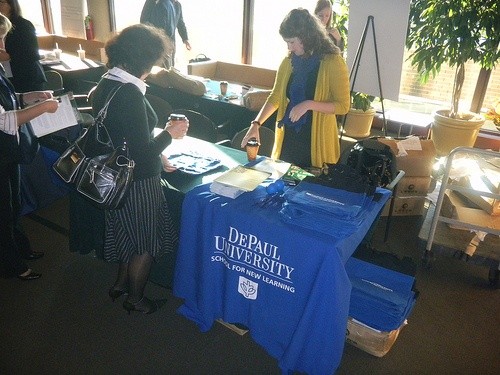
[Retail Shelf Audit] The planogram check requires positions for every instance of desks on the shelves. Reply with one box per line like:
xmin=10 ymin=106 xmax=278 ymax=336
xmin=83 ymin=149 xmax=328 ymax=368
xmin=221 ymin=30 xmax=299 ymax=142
xmin=19 ymin=127 xmax=394 ymax=374
xmin=197 ymin=81 xmax=251 ymax=147
xmin=36 ymin=50 xmax=104 ymax=91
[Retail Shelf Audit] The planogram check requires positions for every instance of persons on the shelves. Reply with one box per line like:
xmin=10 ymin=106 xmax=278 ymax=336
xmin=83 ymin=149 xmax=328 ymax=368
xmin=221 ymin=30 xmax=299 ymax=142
xmin=79 ymin=23 xmax=190 ymax=314
xmin=0 ymin=0 xmax=47 ymax=92
xmin=315 ymin=0 xmax=345 ymax=53
xmin=240 ymin=9 xmax=350 ymax=168
xmin=140 ymin=0 xmax=191 ymax=70
xmin=0 ymin=13 xmax=58 ymax=280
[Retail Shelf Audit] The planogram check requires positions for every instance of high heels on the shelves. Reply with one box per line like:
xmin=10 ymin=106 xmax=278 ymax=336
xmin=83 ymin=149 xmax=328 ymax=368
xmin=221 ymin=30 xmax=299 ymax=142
xmin=108 ymin=287 xmax=129 ymax=302
xmin=122 ymin=296 xmax=168 ymax=315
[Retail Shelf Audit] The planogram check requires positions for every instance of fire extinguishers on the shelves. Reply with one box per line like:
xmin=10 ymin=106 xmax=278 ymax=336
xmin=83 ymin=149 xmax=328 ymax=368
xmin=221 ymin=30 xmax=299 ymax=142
xmin=84 ymin=15 xmax=95 ymax=40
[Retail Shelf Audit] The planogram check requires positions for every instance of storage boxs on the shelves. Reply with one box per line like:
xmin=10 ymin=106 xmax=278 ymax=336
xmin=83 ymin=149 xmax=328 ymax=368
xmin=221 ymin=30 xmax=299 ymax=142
xmin=341 ymin=258 xmax=418 ymax=360
xmin=374 ymin=137 xmax=500 ymax=246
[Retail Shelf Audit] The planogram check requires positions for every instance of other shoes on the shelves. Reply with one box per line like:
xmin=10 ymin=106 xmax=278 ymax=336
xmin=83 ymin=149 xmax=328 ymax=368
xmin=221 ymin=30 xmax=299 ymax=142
xmin=25 ymin=250 xmax=43 ymax=259
xmin=18 ymin=270 xmax=41 ymax=280
xmin=88 ymin=248 xmax=96 ymax=257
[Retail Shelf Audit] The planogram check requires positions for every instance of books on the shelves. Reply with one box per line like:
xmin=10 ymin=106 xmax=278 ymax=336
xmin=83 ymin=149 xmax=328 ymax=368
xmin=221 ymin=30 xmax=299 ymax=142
xmin=210 ymin=164 xmax=272 ymax=199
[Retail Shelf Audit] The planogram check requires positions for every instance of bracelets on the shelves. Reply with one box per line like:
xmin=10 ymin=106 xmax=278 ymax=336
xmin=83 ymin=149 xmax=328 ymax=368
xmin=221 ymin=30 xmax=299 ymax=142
xmin=251 ymin=121 xmax=260 ymax=125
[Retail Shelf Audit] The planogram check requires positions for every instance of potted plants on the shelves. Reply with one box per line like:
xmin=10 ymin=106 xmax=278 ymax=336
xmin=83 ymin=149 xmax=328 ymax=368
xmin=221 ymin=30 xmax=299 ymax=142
xmin=406 ymin=0 xmax=500 ymax=158
xmin=339 ymin=93 xmax=381 ymax=138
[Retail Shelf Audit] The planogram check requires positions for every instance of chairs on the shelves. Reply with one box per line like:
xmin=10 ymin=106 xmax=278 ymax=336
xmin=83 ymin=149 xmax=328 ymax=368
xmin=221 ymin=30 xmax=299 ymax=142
xmin=146 ymin=90 xmax=277 ymax=166
xmin=45 ymin=70 xmax=92 ymax=116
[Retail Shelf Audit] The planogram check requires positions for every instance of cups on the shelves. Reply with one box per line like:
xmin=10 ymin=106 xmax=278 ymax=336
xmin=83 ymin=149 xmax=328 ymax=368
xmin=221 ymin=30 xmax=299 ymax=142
xmin=245 ymin=140 xmax=259 ymax=160
xmin=220 ymin=81 xmax=228 ymax=95
xmin=170 ymin=114 xmax=185 ymax=139
xmin=54 ymin=49 xmax=62 ymax=59
xmin=77 ymin=50 xmax=85 ymax=60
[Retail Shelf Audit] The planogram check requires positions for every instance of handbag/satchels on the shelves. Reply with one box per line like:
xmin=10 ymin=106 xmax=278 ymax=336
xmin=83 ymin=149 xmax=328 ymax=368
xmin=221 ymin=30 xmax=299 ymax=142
xmin=54 ymin=83 xmax=135 ymax=209
xmin=347 ymin=146 xmax=391 ymax=186
xmin=321 ymin=163 xmax=363 ymax=192
